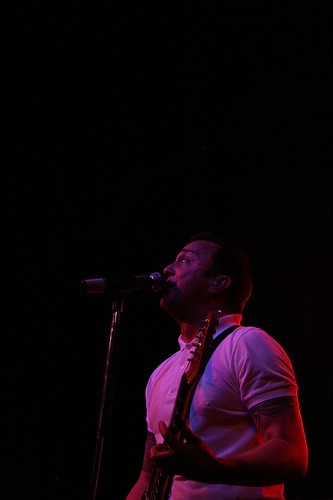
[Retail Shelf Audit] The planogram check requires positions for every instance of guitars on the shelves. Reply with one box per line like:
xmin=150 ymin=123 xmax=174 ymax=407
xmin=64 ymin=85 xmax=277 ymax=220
xmin=138 ymin=309 xmax=219 ymax=500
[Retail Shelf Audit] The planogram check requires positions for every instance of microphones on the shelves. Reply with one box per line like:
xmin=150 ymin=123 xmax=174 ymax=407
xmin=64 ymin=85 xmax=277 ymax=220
xmin=81 ymin=269 xmax=164 ymax=297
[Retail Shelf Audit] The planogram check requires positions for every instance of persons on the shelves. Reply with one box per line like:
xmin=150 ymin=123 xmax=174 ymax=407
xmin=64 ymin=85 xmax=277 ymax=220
xmin=123 ymin=231 xmax=310 ymax=500
